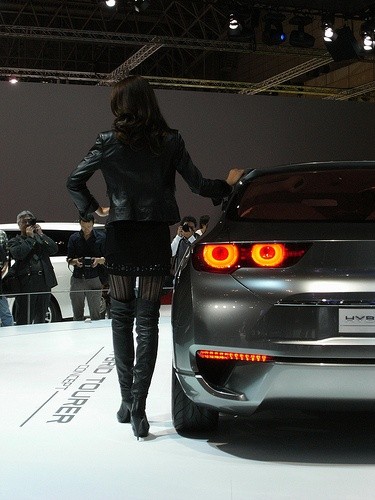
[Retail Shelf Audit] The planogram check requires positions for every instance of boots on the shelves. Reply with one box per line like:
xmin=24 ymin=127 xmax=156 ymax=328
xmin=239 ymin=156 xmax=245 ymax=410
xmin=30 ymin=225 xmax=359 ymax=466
xmin=129 ymin=300 xmax=162 ymax=438
xmin=110 ymin=296 xmax=137 ymax=422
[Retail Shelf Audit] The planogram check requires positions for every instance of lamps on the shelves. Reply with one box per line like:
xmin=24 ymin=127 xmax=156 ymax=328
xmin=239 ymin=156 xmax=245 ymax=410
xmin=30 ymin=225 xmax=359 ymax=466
xmin=271 ymin=28 xmax=286 ymax=41
xmin=360 ymin=20 xmax=375 ymax=51
xmin=228 ymin=12 xmax=241 ymax=30
xmin=321 ymin=13 xmax=334 ymax=42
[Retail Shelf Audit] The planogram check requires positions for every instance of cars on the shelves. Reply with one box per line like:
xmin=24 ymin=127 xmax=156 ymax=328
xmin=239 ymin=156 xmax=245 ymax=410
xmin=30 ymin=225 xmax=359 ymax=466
xmin=0 ymin=221 xmax=139 ymax=323
xmin=171 ymin=159 xmax=375 ymax=438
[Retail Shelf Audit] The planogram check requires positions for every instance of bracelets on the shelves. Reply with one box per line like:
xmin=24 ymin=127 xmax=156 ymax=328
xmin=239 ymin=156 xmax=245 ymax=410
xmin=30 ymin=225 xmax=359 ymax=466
xmin=40 ymin=233 xmax=44 ymax=237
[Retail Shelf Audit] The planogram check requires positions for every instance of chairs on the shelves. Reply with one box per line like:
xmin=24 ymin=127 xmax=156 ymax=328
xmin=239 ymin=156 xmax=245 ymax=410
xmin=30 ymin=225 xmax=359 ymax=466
xmin=342 ymin=188 xmax=375 ymax=222
xmin=240 ymin=192 xmax=319 ymax=219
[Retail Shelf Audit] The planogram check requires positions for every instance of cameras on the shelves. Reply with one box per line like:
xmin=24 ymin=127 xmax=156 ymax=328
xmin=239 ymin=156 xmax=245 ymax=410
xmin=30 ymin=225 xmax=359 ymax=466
xmin=80 ymin=256 xmax=94 ymax=267
xmin=182 ymin=224 xmax=190 ymax=232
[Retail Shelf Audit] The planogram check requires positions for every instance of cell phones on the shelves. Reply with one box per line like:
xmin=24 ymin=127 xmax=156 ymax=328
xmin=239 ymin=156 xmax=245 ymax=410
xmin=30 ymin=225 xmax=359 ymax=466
xmin=30 ymin=219 xmax=36 ymax=227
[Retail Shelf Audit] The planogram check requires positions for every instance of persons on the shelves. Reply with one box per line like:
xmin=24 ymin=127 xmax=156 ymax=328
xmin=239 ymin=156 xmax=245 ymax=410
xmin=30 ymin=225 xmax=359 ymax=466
xmin=195 ymin=215 xmax=210 ymax=237
xmin=9 ymin=210 xmax=58 ymax=325
xmin=67 ymin=78 xmax=242 ymax=441
xmin=0 ymin=230 xmax=14 ymax=326
xmin=67 ymin=212 xmax=106 ymax=321
xmin=170 ymin=216 xmax=200 ymax=273
